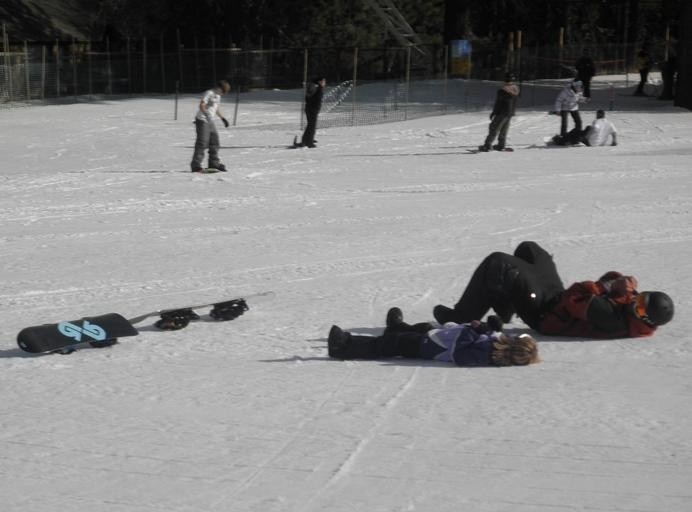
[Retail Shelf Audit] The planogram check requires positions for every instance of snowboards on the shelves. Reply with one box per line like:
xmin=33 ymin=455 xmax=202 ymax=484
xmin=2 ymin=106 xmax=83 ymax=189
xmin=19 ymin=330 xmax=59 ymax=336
xmin=17 ymin=312 xmax=139 ymax=353
xmin=193 ymin=164 xmax=228 ymax=173
xmin=289 ymin=139 xmax=318 ymax=149
xmin=466 ymin=147 xmax=514 ymax=153
xmin=127 ymin=291 xmax=274 ymax=330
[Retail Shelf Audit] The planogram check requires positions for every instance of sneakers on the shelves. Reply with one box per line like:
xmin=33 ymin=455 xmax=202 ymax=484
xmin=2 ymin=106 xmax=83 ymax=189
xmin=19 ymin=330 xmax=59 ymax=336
xmin=493 ymin=144 xmax=505 ymax=151
xmin=477 ymin=144 xmax=492 ymax=152
xmin=191 ymin=162 xmax=229 ymax=173
xmin=430 ymin=300 xmax=467 ymax=332
xmin=382 ymin=303 xmax=407 ymax=339
xmin=492 ymin=297 xmax=515 ymax=325
xmin=327 ymin=322 xmax=349 ymax=363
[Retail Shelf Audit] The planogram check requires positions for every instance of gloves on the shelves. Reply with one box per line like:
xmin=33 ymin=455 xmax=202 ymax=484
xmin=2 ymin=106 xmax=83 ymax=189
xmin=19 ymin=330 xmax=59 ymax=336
xmin=204 ymin=113 xmax=231 ymax=129
xmin=612 ymin=273 xmax=636 ymax=305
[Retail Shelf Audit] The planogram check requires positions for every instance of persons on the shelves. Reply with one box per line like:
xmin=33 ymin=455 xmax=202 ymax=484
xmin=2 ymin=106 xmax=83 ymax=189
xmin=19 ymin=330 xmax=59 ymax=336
xmin=654 ymin=40 xmax=677 ymax=100
xmin=479 ymin=73 xmax=519 ymax=152
xmin=553 ymin=110 xmax=618 ymax=146
xmin=632 ymin=44 xmax=654 ymax=96
xmin=191 ymin=79 xmax=231 ymax=172
xmin=575 ymin=49 xmax=596 ymax=102
xmin=433 ymin=241 xmax=674 ymax=338
xmin=555 ymin=79 xmax=591 ymax=136
xmin=302 ymin=76 xmax=327 ymax=148
xmin=328 ymin=307 xmax=539 ymax=366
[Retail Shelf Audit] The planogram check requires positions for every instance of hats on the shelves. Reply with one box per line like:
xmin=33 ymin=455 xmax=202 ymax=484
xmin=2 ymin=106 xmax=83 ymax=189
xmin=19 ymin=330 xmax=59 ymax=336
xmin=504 ymin=71 xmax=517 ymax=83
xmin=219 ymin=80 xmax=232 ymax=93
xmin=572 ymin=80 xmax=585 ymax=92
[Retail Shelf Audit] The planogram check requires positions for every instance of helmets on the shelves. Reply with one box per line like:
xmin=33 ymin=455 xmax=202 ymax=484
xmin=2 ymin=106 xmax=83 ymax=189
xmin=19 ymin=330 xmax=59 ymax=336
xmin=631 ymin=289 xmax=674 ymax=327
